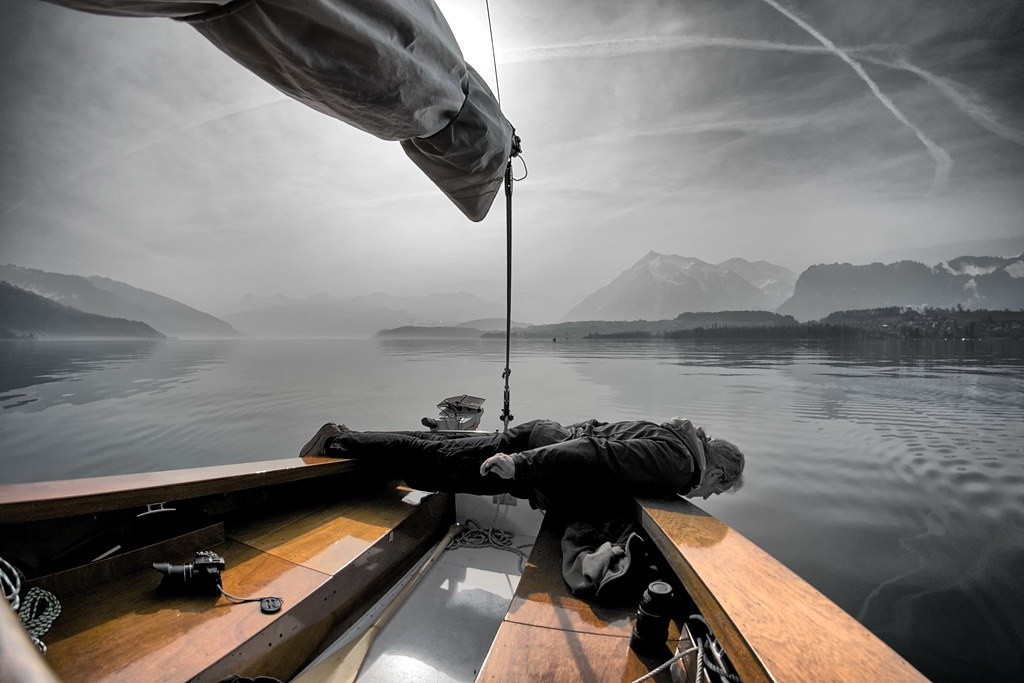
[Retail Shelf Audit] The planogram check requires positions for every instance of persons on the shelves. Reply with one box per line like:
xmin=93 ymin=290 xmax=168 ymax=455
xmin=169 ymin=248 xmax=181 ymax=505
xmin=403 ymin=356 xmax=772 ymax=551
xmin=298 ymin=414 xmax=746 ymax=501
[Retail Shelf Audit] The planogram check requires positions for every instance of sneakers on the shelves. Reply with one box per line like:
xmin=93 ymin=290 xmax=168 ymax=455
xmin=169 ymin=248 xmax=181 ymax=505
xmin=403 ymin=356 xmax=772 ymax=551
xmin=299 ymin=422 xmax=349 ymax=457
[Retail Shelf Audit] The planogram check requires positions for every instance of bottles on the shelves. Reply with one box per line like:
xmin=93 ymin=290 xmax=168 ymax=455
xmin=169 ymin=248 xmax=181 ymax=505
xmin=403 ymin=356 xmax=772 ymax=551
xmin=629 ymin=582 xmax=673 ymax=656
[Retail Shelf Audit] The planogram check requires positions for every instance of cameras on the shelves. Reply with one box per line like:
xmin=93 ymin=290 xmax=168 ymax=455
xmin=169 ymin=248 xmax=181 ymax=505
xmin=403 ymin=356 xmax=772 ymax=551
xmin=153 ymin=551 xmax=225 ymax=600
